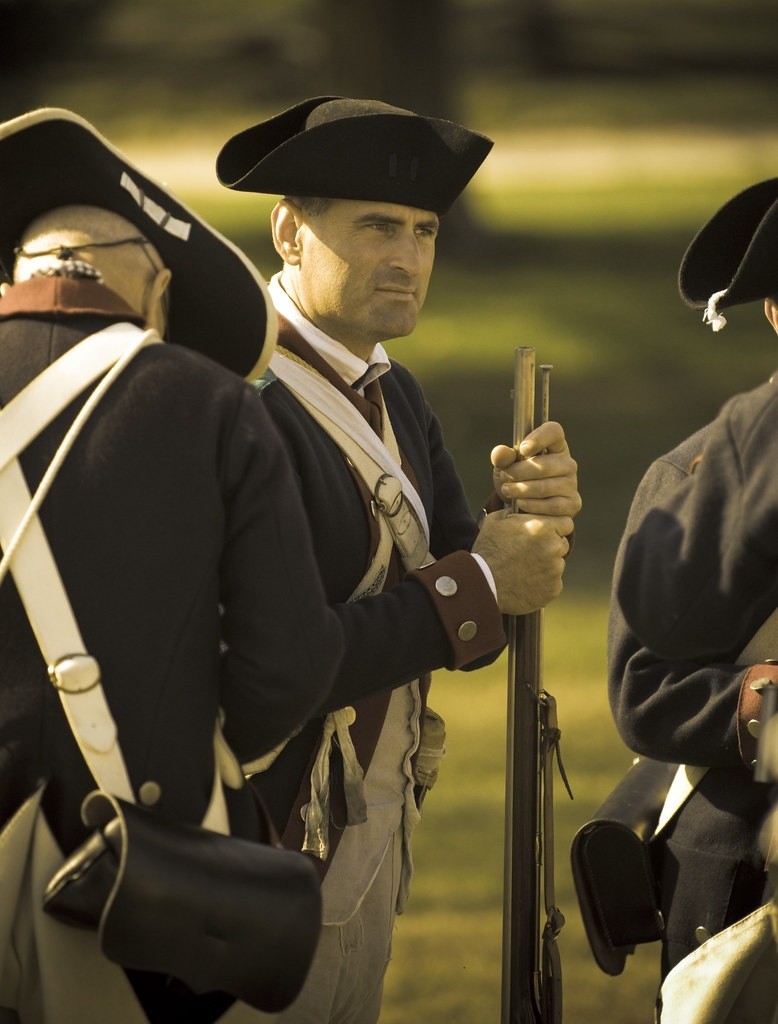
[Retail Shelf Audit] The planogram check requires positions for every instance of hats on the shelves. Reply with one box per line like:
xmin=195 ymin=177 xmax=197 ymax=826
xmin=0 ymin=105 xmax=279 ymax=382
xmin=215 ymin=93 xmax=495 ymax=216
xmin=679 ymin=176 xmax=778 ymax=333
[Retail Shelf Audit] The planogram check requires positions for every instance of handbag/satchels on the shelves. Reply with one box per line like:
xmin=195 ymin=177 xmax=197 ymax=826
xmin=571 ymin=754 xmax=685 ymax=975
xmin=40 ymin=790 xmax=323 ymax=1010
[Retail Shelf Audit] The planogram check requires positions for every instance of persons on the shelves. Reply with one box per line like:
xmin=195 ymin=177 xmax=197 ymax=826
xmin=608 ymin=177 xmax=778 ymax=1024
xmin=1 ymin=106 xmax=344 ymax=1024
xmin=220 ymin=96 xmax=581 ymax=1022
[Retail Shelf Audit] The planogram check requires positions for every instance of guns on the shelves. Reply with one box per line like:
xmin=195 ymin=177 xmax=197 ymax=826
xmin=497 ymin=346 xmax=566 ymax=1024
